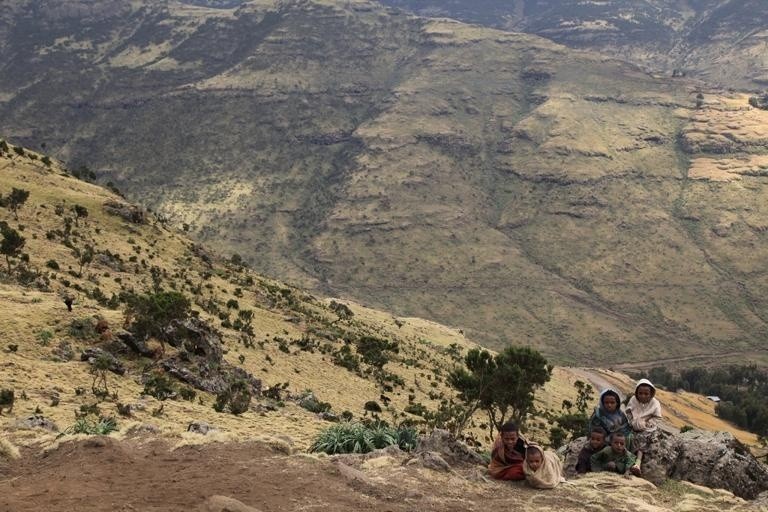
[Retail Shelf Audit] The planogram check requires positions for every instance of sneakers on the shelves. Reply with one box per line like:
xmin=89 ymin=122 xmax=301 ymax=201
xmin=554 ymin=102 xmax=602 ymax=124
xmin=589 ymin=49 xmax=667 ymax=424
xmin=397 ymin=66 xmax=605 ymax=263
xmin=631 ymin=465 xmax=641 ymax=476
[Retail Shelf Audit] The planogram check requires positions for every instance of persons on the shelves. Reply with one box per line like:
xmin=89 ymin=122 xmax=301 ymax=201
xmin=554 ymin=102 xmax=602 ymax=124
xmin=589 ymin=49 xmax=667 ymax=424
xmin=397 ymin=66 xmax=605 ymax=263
xmin=486 ymin=421 xmax=527 ymax=481
xmin=521 ymin=443 xmax=566 ymax=490
xmin=625 ymin=378 xmax=663 ymax=475
xmin=590 ymin=432 xmax=637 ymax=476
xmin=587 ymin=387 xmax=633 ymax=450
xmin=574 ymin=427 xmax=608 ymax=473
